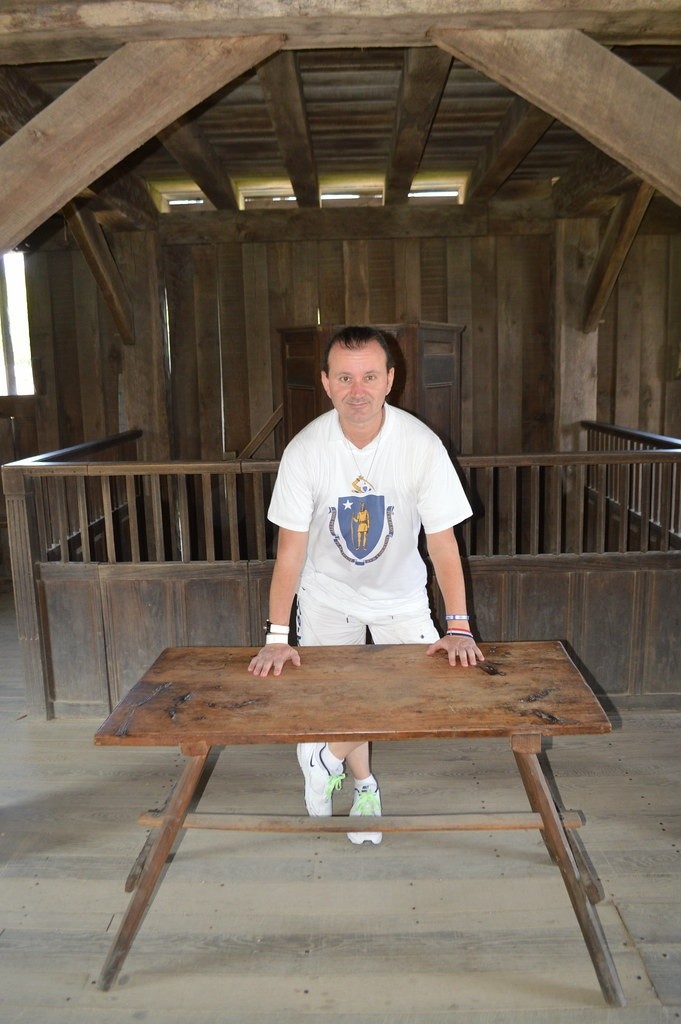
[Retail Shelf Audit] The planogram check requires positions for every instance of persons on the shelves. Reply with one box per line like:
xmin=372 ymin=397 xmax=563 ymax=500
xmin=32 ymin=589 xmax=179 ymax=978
xmin=248 ymin=327 xmax=486 ymax=844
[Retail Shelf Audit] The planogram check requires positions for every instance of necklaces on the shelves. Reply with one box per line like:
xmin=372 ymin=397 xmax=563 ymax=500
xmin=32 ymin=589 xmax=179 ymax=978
xmin=339 ymin=414 xmax=384 ymax=493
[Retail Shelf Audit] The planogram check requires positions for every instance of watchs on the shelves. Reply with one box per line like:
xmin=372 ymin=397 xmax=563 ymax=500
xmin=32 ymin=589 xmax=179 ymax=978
xmin=263 ymin=619 xmax=290 ymax=635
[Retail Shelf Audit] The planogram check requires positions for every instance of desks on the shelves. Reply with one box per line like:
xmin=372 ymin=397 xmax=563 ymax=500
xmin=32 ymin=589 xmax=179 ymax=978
xmin=91 ymin=639 xmax=626 ymax=1007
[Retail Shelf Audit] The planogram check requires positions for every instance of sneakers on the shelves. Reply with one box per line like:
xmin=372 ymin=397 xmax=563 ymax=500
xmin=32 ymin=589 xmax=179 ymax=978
xmin=297 ymin=742 xmax=345 ymax=816
xmin=347 ymin=787 xmax=383 ymax=848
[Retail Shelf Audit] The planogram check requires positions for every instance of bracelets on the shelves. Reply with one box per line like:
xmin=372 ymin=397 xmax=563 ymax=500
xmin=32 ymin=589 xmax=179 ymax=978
xmin=265 ymin=635 xmax=288 ymax=645
xmin=445 ymin=628 xmax=474 ymax=638
xmin=446 ymin=615 xmax=469 ymax=620
